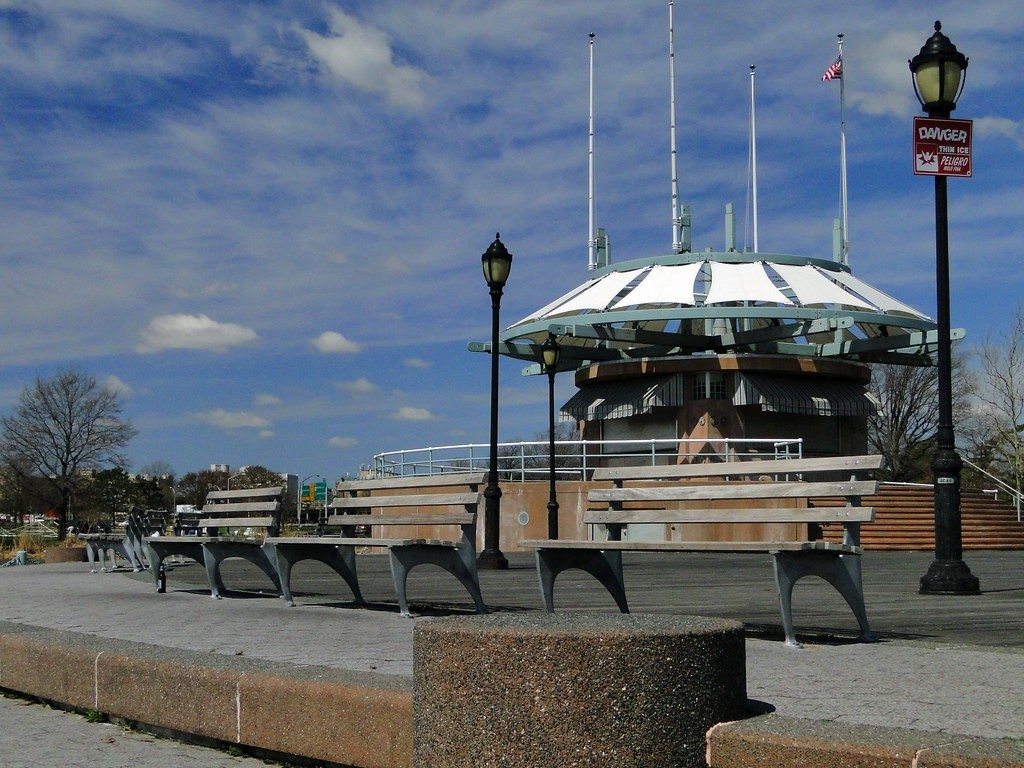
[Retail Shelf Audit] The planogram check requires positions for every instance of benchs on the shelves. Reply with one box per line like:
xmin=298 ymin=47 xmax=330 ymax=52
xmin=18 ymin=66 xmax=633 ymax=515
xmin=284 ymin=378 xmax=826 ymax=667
xmin=142 ymin=486 xmax=287 ymax=599
xmin=265 ymin=472 xmax=487 ymax=618
xmin=76 ymin=505 xmax=146 ymax=572
xmin=141 ymin=508 xmax=168 ymax=569
xmin=517 ymin=454 xmax=886 ymax=646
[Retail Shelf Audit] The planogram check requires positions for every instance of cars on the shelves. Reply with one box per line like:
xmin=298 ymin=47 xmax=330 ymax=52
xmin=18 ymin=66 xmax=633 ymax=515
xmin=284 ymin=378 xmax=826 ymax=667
xmin=119 ymin=521 xmax=130 ymax=527
xmin=174 ymin=512 xmax=208 ymax=536
xmin=355 ymin=526 xmax=367 ymax=532
xmin=111 ymin=517 xmax=124 ymax=526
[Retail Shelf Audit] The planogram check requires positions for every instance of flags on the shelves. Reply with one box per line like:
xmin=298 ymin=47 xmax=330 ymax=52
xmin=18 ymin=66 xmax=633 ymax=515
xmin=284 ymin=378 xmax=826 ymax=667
xmin=821 ymin=54 xmax=842 ymax=81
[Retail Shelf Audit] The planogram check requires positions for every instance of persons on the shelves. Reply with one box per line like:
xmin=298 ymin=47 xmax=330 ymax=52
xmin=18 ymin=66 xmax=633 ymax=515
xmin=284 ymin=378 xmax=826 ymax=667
xmin=80 ymin=521 xmax=89 ymax=533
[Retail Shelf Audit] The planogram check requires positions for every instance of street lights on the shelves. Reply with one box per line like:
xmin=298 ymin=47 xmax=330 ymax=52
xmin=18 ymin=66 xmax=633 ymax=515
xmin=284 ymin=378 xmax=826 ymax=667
xmin=170 ymin=487 xmax=176 ymax=524
xmin=540 ymin=332 xmax=562 ymax=540
xmin=298 ymin=475 xmax=319 ymax=530
xmin=227 ymin=473 xmax=246 ymax=534
xmin=247 ymin=484 xmax=262 ymax=518
xmin=909 ymin=21 xmax=983 ymax=595
xmin=477 ymin=231 xmax=514 ymax=570
